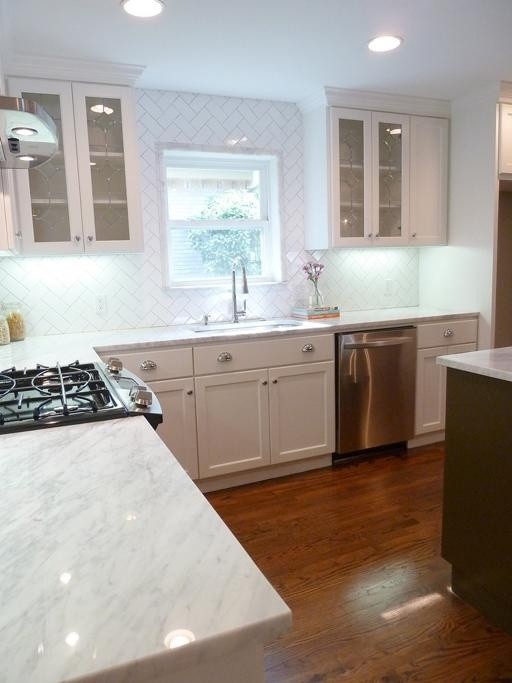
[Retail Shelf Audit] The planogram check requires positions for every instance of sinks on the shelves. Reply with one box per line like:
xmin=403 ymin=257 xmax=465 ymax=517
xmin=187 ymin=320 xmax=305 ymax=333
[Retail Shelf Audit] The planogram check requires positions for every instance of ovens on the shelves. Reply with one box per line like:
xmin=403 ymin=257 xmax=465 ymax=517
xmin=333 ymin=326 xmax=417 ymax=469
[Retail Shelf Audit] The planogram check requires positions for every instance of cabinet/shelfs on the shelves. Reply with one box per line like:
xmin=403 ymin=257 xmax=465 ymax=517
xmin=297 ymin=85 xmax=451 ymax=249
xmin=1 ymin=53 xmax=146 ymax=255
xmin=99 ymin=318 xmax=480 ymax=502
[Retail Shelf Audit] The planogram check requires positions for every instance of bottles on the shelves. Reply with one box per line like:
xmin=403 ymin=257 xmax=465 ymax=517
xmin=0 ymin=301 xmax=25 ymax=346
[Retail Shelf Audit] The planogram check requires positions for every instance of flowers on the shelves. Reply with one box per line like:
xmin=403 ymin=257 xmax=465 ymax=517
xmin=303 ymin=260 xmax=327 ymax=304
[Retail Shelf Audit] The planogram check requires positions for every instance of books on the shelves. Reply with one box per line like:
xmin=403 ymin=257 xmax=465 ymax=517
xmin=292 ymin=305 xmax=340 ymax=319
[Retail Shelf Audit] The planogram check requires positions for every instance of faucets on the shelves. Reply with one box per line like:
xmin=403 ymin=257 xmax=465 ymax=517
xmin=231 ymin=256 xmax=248 ymax=320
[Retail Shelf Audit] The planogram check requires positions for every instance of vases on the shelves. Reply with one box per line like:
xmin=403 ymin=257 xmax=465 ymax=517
xmin=308 ymin=290 xmax=324 ymax=310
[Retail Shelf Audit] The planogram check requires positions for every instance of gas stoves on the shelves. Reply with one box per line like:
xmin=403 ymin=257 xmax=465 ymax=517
xmin=0 ymin=357 xmax=163 ymax=435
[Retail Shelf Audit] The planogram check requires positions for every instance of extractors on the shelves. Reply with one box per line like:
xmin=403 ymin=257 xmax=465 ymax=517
xmin=0 ymin=97 xmax=59 ymax=169
